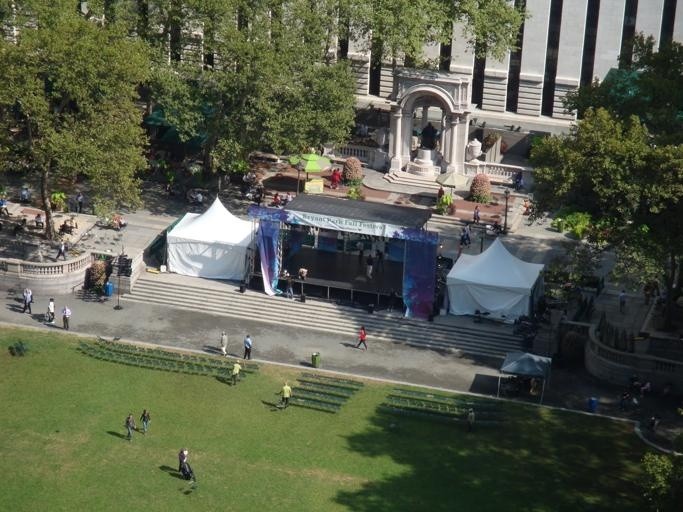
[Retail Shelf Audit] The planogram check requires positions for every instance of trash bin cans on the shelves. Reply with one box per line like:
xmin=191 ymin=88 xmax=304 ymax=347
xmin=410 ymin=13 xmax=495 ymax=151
xmin=588 ymin=396 xmax=598 ymax=409
xmin=104 ymin=282 xmax=113 ymax=296
xmin=312 ymin=352 xmax=321 ymax=368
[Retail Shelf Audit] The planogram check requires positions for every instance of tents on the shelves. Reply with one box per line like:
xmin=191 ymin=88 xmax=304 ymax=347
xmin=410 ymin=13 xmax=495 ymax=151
xmin=167 ymin=197 xmax=259 ymax=281
xmin=446 ymin=237 xmax=546 ymax=325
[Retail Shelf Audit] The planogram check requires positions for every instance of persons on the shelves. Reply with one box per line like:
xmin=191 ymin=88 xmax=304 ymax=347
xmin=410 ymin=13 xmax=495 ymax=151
xmin=514 ymin=293 xmax=635 ymax=355
xmin=220 ymin=331 xmax=252 ymax=385
xmin=188 ymin=171 xmax=292 ymax=205
xmin=459 ymin=205 xmax=481 ymax=250
xmin=330 ymin=167 xmax=345 ymax=188
xmin=179 ymin=448 xmax=196 ymax=481
xmin=366 ymin=254 xmax=376 ymax=280
xmin=467 ymin=408 xmax=476 ymax=434
xmin=337 ymin=232 xmax=386 ymax=260
xmin=127 ymin=409 xmax=151 ymax=439
xmin=22 ymin=289 xmax=72 ymax=331
xmin=619 ymin=374 xmax=675 ymax=410
xmin=354 ymin=326 xmax=368 ymax=349
xmin=285 ymin=267 xmax=308 ymax=298
xmin=276 ymin=381 xmax=292 ymax=406
xmin=2 ymin=191 xmax=84 ymax=262
xmin=619 ymin=289 xmax=627 ymax=312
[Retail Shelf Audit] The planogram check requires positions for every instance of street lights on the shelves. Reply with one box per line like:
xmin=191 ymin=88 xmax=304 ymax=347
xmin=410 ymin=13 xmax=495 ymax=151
xmin=294 ymin=159 xmax=302 ymax=196
xmin=479 ymin=227 xmax=486 ymax=252
xmin=503 ymin=187 xmax=511 ymax=238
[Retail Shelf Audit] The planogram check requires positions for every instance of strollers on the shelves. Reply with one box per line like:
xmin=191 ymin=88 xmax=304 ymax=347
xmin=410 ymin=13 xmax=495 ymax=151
xmin=180 ymin=462 xmax=199 ymax=483
